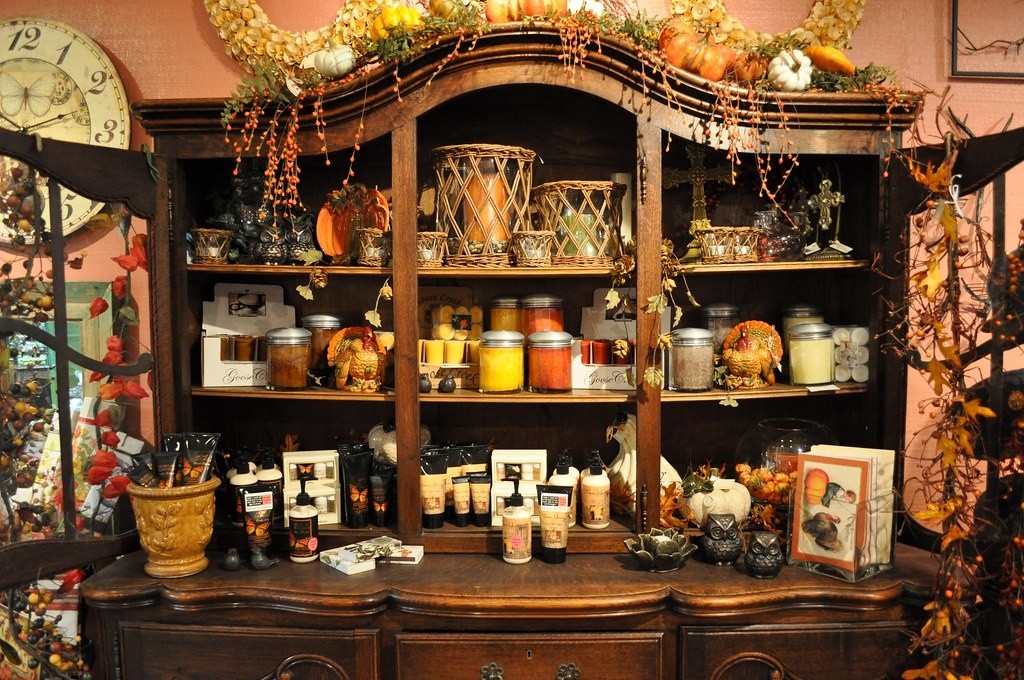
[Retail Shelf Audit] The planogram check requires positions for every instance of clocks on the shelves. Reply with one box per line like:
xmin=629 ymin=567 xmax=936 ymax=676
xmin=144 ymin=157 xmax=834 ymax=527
xmin=0 ymin=13 xmax=131 ymax=250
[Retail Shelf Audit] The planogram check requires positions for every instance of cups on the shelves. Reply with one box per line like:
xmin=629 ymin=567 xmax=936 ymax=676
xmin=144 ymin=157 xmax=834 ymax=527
xmin=220 ymin=334 xmax=268 ymax=360
xmin=581 ymin=339 xmax=632 ymax=365
xmin=418 ymin=339 xmax=480 ymax=364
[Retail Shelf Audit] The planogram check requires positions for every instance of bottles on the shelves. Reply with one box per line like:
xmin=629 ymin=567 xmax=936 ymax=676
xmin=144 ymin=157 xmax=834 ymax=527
xmin=265 ymin=326 xmax=313 ymax=388
xmin=788 ymin=323 xmax=834 ymax=384
xmin=783 ymin=306 xmax=824 ymax=354
xmin=478 ymin=329 xmax=525 ymax=393
xmin=668 ymin=328 xmax=714 ymax=391
xmin=302 ymin=315 xmax=341 ymax=378
xmin=702 ymin=303 xmax=742 ymax=353
xmin=522 ymin=295 xmax=564 ymax=330
xmin=491 ymin=299 xmax=521 ymax=330
xmin=528 ymin=330 xmax=572 ymax=392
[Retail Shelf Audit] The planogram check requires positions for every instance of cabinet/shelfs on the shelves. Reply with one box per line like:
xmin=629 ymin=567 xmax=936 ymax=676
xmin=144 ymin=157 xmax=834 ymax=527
xmin=134 ymin=22 xmax=929 ymax=556
xmin=76 ymin=543 xmax=981 ymax=680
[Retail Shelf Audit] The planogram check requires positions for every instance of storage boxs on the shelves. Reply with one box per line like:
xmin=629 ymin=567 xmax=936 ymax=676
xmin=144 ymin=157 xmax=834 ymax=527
xmin=31 ymin=396 xmax=144 ymax=535
xmin=28 ymin=578 xmax=81 ymax=647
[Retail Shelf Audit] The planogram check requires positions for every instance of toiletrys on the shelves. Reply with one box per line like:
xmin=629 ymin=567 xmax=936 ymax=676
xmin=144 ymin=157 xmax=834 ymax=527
xmin=126 ymin=432 xmax=611 ymax=564
